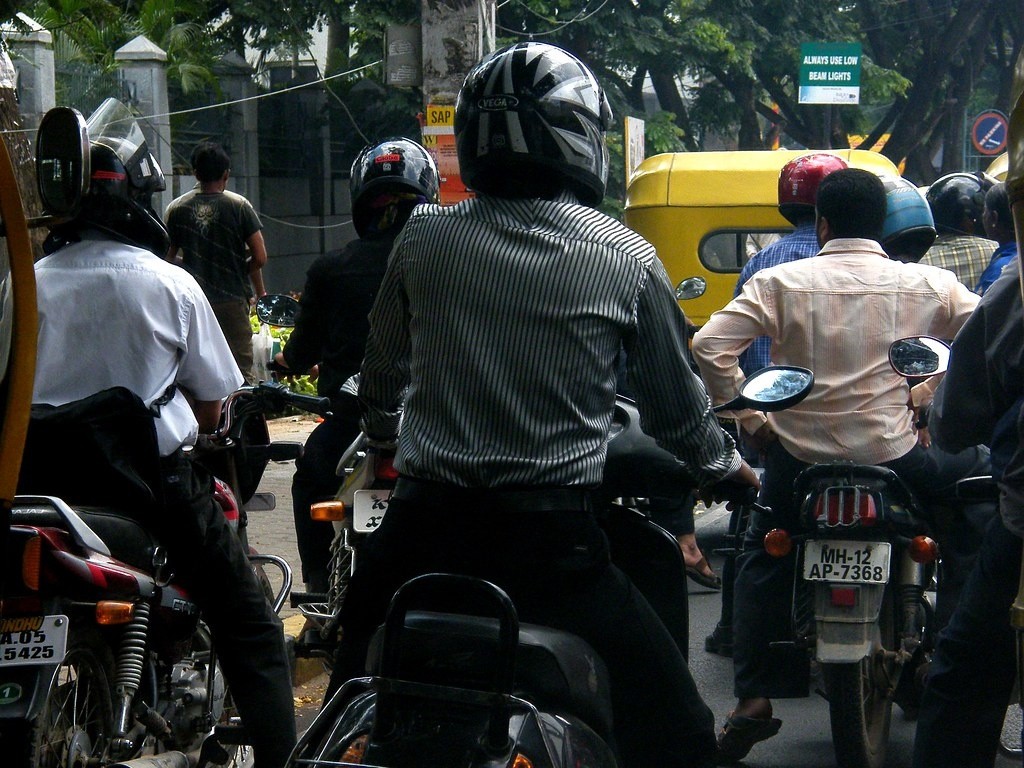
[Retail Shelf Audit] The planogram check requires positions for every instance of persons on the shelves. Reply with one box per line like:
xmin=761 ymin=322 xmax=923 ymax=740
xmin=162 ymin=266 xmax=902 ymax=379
xmin=693 ymin=154 xmax=1024 ymax=768
xmin=163 ymin=141 xmax=266 ymax=387
xmin=322 ymin=41 xmax=761 ymax=768
xmin=272 ymin=137 xmax=441 ymax=656
xmin=1 ymin=99 xmax=296 ymax=767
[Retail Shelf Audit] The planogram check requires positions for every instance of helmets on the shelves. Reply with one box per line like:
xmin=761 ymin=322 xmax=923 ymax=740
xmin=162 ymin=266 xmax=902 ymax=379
xmin=778 ymin=153 xmax=849 ymax=227
xmin=49 ymin=136 xmax=170 ymax=260
xmin=453 ymin=42 xmax=617 ymax=209
xmin=878 ymin=176 xmax=937 ymax=263
xmin=925 ymin=172 xmax=988 ymax=237
xmin=349 ymin=136 xmax=440 ymax=239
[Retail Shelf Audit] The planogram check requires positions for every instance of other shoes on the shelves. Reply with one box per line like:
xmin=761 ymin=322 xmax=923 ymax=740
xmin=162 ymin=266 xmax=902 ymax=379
xmin=705 ymin=624 xmax=735 ymax=656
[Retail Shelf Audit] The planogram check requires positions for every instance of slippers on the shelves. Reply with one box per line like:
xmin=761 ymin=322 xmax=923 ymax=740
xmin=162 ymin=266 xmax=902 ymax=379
xmin=684 ymin=556 xmax=721 ymax=590
xmin=717 ymin=711 xmax=781 ymax=761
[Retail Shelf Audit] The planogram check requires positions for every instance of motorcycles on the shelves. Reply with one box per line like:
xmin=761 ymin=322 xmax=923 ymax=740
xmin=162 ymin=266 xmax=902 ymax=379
xmin=1 ymin=378 xmax=334 ymax=767
xmin=286 ymin=364 xmax=814 ymax=767
xmin=0 ymin=109 xmax=90 ymax=602
xmin=252 ymin=148 xmax=1024 ymax=767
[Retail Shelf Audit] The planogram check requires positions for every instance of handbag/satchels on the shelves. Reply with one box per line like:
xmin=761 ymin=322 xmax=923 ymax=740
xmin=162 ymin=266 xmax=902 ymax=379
xmin=15 ymin=386 xmax=164 ymax=511
